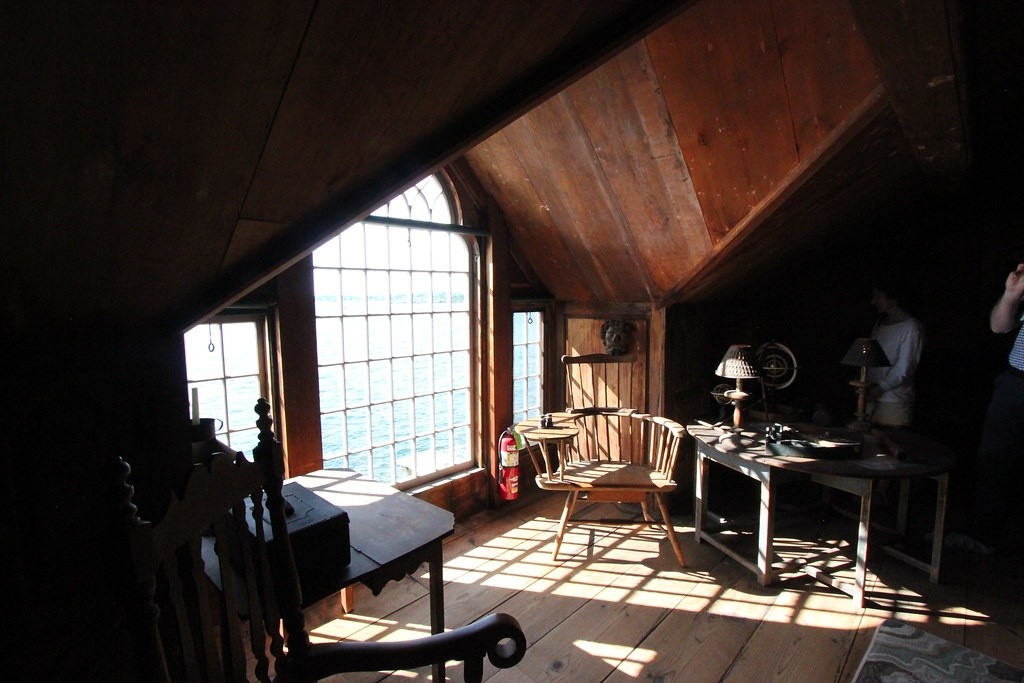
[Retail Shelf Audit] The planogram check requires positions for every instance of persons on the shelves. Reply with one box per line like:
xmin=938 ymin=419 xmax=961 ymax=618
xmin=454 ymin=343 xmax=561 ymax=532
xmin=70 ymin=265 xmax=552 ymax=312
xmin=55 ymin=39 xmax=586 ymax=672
xmin=600 ymin=321 xmax=635 ymax=356
xmin=980 ymin=264 xmax=1024 ymax=519
xmin=866 ymin=285 xmax=925 ymax=428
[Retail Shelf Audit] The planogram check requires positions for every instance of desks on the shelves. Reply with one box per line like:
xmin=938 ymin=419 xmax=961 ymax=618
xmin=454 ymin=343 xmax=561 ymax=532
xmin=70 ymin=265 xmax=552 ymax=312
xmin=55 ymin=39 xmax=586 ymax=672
xmin=689 ymin=424 xmax=947 ymax=609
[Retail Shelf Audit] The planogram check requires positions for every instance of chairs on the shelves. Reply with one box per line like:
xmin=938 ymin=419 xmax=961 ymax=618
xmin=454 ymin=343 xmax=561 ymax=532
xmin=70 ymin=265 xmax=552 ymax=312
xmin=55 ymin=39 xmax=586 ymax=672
xmin=113 ymin=395 xmax=526 ymax=683
xmin=532 ymin=350 xmax=685 ymax=571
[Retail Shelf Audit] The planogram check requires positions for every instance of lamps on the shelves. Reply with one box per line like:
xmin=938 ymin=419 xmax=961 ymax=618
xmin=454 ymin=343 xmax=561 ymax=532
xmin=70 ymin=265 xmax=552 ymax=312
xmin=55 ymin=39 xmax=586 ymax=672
xmin=714 ymin=343 xmax=759 ymax=436
xmin=837 ymin=336 xmax=892 ymax=435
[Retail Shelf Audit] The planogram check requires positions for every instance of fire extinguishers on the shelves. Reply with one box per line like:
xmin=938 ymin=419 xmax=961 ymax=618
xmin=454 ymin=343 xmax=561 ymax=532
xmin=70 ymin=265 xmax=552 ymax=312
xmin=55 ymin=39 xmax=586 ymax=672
xmin=497 ymin=422 xmax=520 ymax=501
